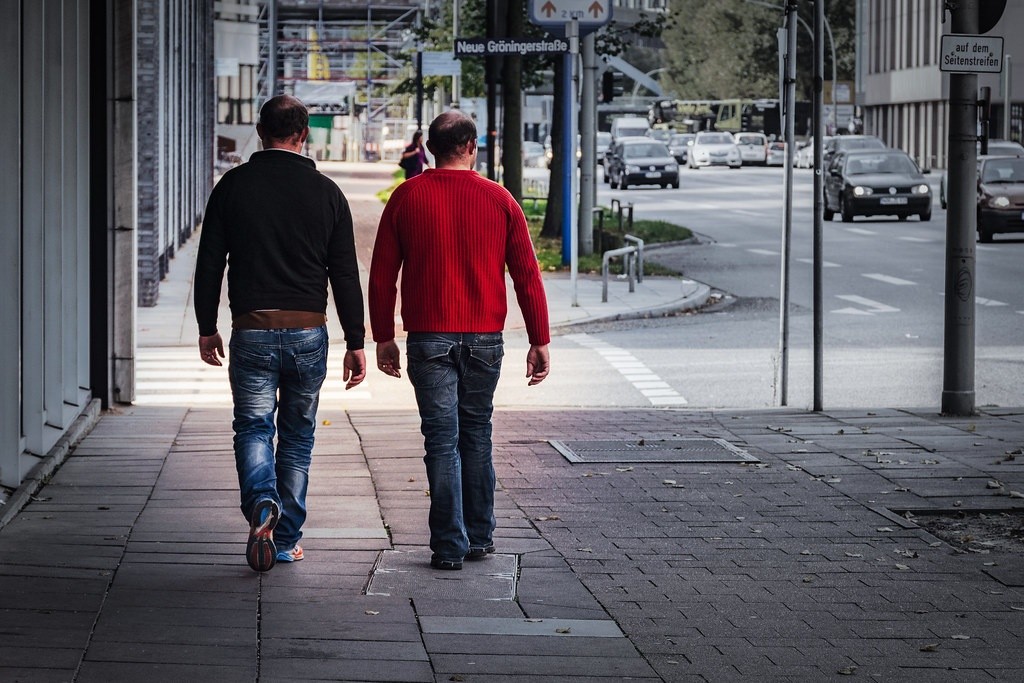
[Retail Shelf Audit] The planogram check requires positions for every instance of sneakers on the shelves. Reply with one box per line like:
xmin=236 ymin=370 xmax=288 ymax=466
xmin=277 ymin=544 xmax=303 ymax=562
xmin=246 ymin=498 xmax=281 ymax=572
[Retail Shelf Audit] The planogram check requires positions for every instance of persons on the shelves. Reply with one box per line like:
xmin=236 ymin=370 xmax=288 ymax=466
xmin=194 ymin=95 xmax=366 ymax=570
xmin=403 ymin=130 xmax=430 ymax=179
xmin=369 ymin=110 xmax=549 ymax=568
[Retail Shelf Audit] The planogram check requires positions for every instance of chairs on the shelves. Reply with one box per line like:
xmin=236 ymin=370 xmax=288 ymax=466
xmin=848 ymin=161 xmax=861 ymax=172
xmin=878 ymin=160 xmax=888 ymax=173
xmin=986 ymin=169 xmax=1001 ymax=180
xmin=1010 ymin=167 xmax=1024 ymax=179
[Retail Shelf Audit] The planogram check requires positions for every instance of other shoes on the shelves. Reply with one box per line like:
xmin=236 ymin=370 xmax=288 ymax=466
xmin=467 ymin=543 xmax=497 ymax=558
xmin=430 ymin=557 xmax=463 ymax=571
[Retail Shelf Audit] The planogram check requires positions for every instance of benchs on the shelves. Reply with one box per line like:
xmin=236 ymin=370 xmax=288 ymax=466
xmin=862 ymin=163 xmax=879 ymax=172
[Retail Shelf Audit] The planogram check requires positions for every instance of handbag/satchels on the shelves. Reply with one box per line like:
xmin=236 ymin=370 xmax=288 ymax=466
xmin=399 ymin=155 xmax=413 ymax=170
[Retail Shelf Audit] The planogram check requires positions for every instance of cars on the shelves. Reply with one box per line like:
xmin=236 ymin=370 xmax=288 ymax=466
xmin=524 ymin=117 xmax=834 ymax=170
xmin=938 ymin=137 xmax=1024 ymax=210
xmin=608 ymin=140 xmax=681 ymax=191
xmin=686 ymin=131 xmax=742 ymax=170
xmin=601 ymin=135 xmax=655 ymax=183
xmin=979 ymin=154 xmax=1024 ymax=244
xmin=823 ymin=147 xmax=935 ymax=224
xmin=822 ymin=135 xmax=890 ymax=170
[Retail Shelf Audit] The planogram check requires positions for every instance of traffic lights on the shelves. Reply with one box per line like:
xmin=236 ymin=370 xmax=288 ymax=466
xmin=601 ymin=70 xmax=626 ymax=104
